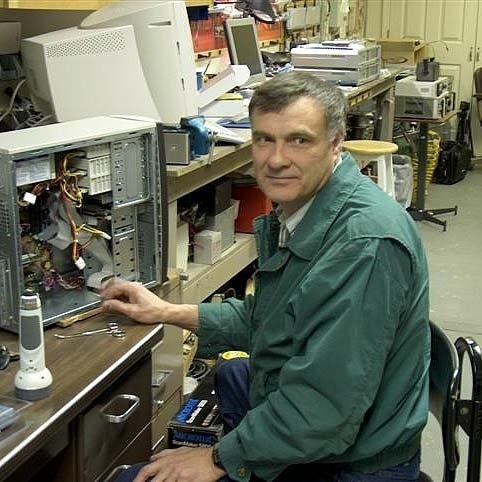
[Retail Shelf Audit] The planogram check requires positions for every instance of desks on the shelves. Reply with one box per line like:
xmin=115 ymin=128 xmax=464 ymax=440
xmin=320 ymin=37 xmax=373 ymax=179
xmin=0 ymin=315 xmax=164 ymax=482
xmin=348 ymin=100 xmax=459 ymax=232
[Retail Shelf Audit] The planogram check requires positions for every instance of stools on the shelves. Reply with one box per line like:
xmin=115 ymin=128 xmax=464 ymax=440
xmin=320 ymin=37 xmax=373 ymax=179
xmin=343 ymin=139 xmax=398 ymax=201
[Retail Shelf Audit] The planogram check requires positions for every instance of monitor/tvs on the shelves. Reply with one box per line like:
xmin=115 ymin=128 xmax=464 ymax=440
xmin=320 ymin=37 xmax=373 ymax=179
xmin=22 ymin=0 xmax=203 ymax=127
xmin=223 ymin=17 xmax=267 ymax=87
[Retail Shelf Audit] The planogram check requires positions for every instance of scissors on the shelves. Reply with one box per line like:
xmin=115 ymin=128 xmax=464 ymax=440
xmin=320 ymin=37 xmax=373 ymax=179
xmin=53 ymin=322 xmax=127 ymax=339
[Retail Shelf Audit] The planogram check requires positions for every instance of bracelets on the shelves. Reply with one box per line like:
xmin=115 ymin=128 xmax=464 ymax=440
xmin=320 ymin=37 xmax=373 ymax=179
xmin=212 ymin=444 xmax=228 ymax=473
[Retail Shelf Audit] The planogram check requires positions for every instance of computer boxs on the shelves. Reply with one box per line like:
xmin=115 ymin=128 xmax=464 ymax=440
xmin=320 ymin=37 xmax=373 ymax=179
xmin=0 ymin=112 xmax=169 ymax=335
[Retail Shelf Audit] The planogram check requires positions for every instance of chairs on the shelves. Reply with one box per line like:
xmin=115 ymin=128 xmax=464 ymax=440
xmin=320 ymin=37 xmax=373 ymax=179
xmin=419 ymin=320 xmax=482 ymax=482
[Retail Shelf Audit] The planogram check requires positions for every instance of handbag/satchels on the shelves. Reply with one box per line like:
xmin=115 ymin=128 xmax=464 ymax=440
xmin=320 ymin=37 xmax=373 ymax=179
xmin=437 ymin=141 xmax=466 ymax=184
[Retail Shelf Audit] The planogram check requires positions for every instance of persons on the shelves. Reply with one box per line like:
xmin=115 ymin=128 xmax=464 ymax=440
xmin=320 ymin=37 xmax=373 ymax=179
xmin=101 ymin=70 xmax=431 ymax=482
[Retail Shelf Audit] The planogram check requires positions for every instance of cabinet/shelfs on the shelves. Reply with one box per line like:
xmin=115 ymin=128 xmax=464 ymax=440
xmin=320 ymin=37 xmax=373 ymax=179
xmin=152 ymin=75 xmax=398 ymax=458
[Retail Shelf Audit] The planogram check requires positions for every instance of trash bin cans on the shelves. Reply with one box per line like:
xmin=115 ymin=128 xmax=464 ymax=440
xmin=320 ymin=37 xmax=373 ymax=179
xmin=392 ymin=154 xmax=414 ymax=210
